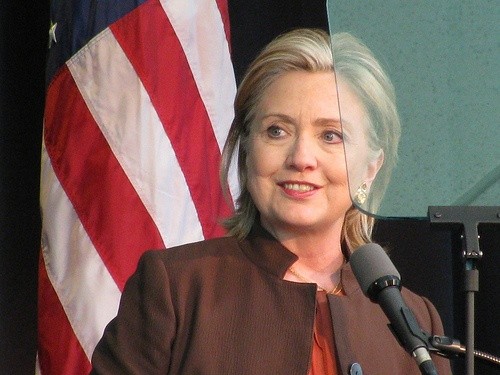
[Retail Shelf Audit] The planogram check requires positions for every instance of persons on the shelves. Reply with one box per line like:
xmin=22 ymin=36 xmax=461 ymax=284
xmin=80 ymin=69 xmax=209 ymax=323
xmin=90 ymin=28 xmax=452 ymax=375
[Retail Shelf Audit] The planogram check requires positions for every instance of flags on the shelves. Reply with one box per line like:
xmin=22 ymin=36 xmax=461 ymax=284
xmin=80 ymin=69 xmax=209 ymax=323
xmin=35 ymin=0 xmax=241 ymax=375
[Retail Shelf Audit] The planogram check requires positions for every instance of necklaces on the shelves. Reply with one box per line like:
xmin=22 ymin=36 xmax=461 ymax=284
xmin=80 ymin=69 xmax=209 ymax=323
xmin=289 ymin=259 xmax=347 ymax=295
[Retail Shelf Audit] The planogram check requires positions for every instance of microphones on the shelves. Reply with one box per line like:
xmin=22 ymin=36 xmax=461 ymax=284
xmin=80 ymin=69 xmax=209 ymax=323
xmin=349 ymin=243 xmax=438 ymax=375
